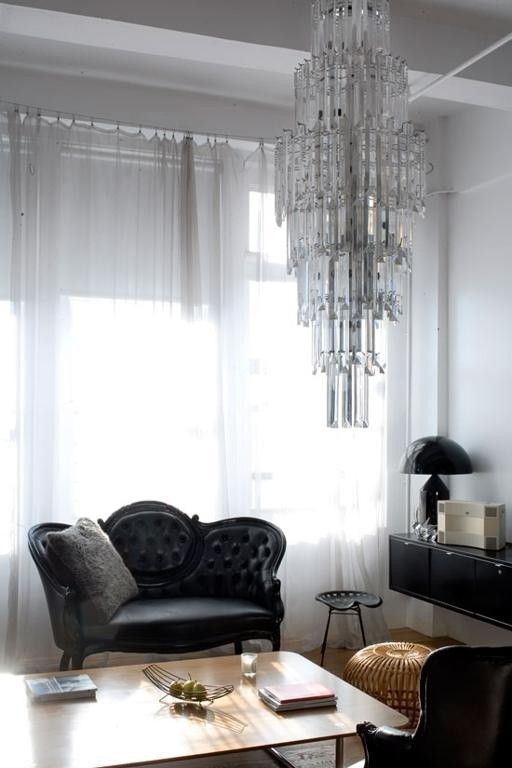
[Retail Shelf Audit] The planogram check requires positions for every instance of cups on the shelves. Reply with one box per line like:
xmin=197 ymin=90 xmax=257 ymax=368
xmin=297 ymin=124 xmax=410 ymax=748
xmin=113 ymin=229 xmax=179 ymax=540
xmin=241 ymin=652 xmax=258 ymax=678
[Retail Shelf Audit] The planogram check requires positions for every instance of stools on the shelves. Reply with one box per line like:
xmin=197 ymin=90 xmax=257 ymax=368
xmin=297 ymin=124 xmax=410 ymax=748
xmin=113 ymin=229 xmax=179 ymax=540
xmin=315 ymin=589 xmax=383 ymax=667
xmin=343 ymin=641 xmax=437 ymax=730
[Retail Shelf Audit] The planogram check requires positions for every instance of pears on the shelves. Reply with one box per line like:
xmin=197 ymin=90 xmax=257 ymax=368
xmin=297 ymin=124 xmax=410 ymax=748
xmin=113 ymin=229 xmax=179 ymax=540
xmin=169 ymin=677 xmax=184 ymax=696
xmin=193 ymin=679 xmax=206 ymax=699
xmin=183 ymin=672 xmax=197 ymax=699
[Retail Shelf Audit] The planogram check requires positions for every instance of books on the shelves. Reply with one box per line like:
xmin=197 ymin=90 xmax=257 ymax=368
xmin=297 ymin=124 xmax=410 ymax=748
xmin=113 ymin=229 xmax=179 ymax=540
xmin=257 ymin=681 xmax=338 ymax=713
xmin=24 ymin=673 xmax=98 ymax=703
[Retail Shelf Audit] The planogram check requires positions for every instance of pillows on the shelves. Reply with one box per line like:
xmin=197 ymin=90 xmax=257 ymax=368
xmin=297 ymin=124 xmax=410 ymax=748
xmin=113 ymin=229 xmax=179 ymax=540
xmin=45 ymin=517 xmax=139 ymax=625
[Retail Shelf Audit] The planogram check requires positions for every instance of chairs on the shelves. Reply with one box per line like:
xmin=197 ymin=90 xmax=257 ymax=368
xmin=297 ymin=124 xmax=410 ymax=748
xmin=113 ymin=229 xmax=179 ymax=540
xmin=356 ymin=645 xmax=512 ymax=768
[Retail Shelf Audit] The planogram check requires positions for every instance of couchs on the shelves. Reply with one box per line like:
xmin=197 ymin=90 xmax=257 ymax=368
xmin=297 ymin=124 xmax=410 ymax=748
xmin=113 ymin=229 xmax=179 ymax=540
xmin=27 ymin=500 xmax=286 ymax=672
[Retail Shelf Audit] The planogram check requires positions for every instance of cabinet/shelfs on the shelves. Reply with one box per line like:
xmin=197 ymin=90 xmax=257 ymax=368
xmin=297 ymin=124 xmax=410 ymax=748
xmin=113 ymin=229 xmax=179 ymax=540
xmin=388 ymin=532 xmax=512 ymax=632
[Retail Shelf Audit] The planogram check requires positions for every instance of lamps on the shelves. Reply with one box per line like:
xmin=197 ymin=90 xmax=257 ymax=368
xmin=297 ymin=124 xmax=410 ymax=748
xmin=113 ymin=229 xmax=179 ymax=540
xmin=398 ymin=436 xmax=472 ymax=525
xmin=276 ymin=0 xmax=427 ymax=427
